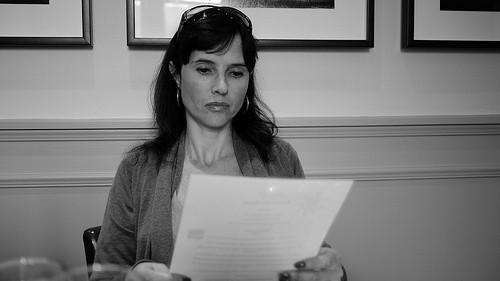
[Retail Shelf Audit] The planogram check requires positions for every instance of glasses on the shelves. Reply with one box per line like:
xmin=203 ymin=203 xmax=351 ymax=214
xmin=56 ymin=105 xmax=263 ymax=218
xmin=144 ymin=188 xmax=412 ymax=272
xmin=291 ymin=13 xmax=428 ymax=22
xmin=177 ymin=5 xmax=252 ymax=40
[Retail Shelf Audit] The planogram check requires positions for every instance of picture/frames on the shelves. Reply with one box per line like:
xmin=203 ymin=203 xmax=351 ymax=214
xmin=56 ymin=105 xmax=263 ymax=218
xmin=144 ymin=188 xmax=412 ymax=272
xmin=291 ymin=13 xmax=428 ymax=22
xmin=400 ymin=0 xmax=500 ymax=53
xmin=0 ymin=0 xmax=92 ymax=50
xmin=125 ymin=0 xmax=375 ymax=50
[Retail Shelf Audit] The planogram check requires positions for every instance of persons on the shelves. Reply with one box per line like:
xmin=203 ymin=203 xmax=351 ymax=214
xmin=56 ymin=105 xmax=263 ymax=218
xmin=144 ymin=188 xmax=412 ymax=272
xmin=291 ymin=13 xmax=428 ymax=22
xmin=91 ymin=4 xmax=348 ymax=281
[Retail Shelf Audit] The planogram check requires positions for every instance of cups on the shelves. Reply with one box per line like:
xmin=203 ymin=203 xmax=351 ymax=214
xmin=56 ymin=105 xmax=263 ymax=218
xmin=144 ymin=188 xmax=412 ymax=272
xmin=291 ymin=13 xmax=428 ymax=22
xmin=0 ymin=257 xmax=139 ymax=281
